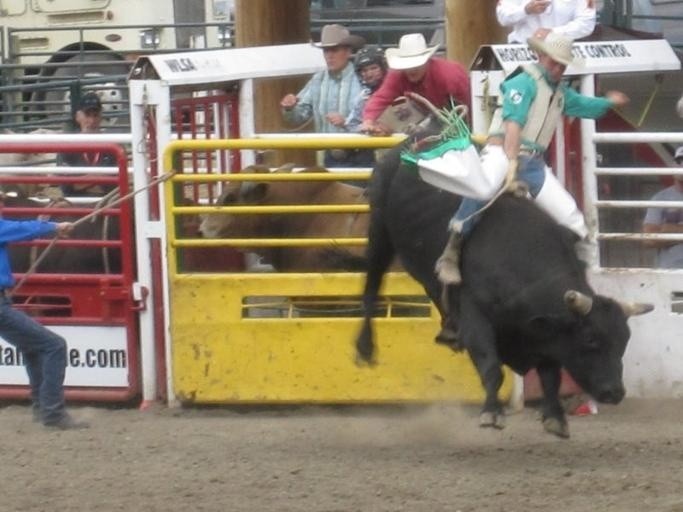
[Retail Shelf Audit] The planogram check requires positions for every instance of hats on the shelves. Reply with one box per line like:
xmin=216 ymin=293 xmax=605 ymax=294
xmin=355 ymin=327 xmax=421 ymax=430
xmin=307 ymin=23 xmax=368 ymax=50
xmin=76 ymin=91 xmax=102 ymax=111
xmin=674 ymin=146 xmax=683 ymax=159
xmin=383 ymin=32 xmax=444 ymax=72
xmin=523 ymin=29 xmax=591 ymax=69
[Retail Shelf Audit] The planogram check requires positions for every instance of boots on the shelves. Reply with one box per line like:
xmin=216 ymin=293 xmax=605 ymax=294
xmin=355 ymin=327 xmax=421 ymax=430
xmin=432 ymin=227 xmax=467 ymax=287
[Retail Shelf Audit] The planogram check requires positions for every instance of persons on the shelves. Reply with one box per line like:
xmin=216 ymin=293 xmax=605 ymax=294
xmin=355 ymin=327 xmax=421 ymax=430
xmin=344 ymin=43 xmax=432 ymax=136
xmin=675 ymin=95 xmax=683 ymax=124
xmin=359 ymin=32 xmax=471 ymax=138
xmin=0 ymin=190 xmax=85 ymax=429
xmin=594 ymin=144 xmax=618 ymax=199
xmin=641 ymin=147 xmax=683 ymax=271
xmin=279 ymin=23 xmax=376 ymax=189
xmin=434 ymin=30 xmax=630 ymax=286
xmin=496 ymin=0 xmax=597 ymax=45
xmin=53 ymin=93 xmax=119 ymax=275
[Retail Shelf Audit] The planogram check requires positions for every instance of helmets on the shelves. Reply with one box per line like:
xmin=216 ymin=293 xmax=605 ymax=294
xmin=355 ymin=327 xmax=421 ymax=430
xmin=350 ymin=44 xmax=388 ymax=91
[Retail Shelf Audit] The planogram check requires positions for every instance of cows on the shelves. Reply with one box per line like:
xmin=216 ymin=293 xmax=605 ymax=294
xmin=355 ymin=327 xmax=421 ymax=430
xmin=3 ymin=195 xmax=122 ymax=317
xmin=352 ymin=93 xmax=655 ymax=440
xmin=193 ymin=161 xmax=431 ymax=318
xmin=0 ymin=127 xmax=66 ymax=196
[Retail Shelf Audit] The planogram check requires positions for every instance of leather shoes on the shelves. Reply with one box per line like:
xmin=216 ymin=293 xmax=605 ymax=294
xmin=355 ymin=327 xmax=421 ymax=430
xmin=43 ymin=411 xmax=90 ymax=430
xmin=30 ymin=405 xmax=43 ymax=422
